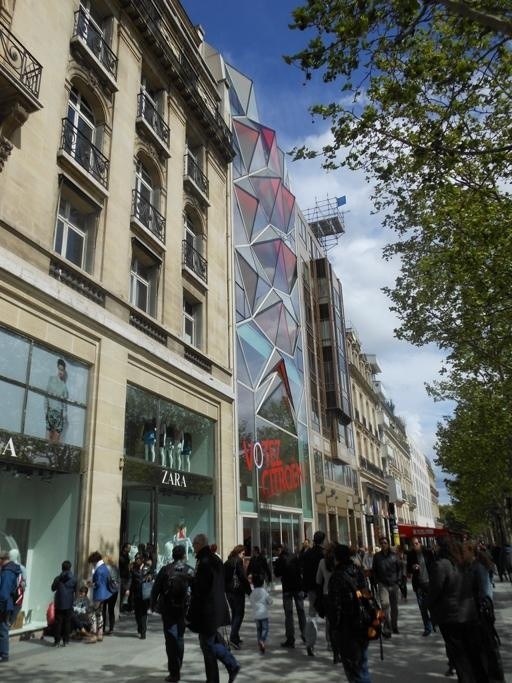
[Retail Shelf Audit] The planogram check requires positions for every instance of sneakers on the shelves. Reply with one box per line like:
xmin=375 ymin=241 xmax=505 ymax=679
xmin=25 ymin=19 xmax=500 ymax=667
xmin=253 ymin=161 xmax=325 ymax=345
xmin=136 ymin=628 xmax=147 ymax=639
xmin=0 ymin=656 xmax=9 ymax=661
xmin=71 ymin=631 xmax=114 ymax=644
xmin=227 ymin=664 xmax=241 ymax=683
xmin=50 ymin=641 xmax=68 ymax=648
xmin=491 ymin=576 xmax=512 ymax=588
xmin=164 ymin=674 xmax=180 ymax=683
xmin=229 ymin=636 xmax=244 ymax=650
xmin=381 ymin=627 xmax=400 ymax=639
xmin=422 ymin=624 xmax=440 ymax=637
xmin=256 ymin=639 xmax=267 ymax=654
xmin=279 ymin=638 xmax=296 ymax=648
xmin=305 ymin=644 xmax=314 ymax=657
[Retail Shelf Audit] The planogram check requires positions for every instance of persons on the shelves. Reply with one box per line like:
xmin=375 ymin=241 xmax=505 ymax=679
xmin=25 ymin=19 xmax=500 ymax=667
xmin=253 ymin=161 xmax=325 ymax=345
xmin=141 ymin=417 xmax=193 ymax=473
xmin=175 ymin=516 xmax=194 ymax=561
xmin=44 ymin=360 xmax=69 ymax=442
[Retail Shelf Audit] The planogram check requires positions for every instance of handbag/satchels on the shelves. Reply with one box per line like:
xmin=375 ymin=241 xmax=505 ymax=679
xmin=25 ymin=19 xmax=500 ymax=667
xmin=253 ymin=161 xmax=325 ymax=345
xmin=473 ymin=595 xmax=497 ymax=630
xmin=106 ymin=564 xmax=122 ymax=592
xmin=14 ymin=572 xmax=24 ymax=605
xmin=230 ymin=557 xmax=243 ymax=593
xmin=141 ymin=575 xmax=155 ymax=600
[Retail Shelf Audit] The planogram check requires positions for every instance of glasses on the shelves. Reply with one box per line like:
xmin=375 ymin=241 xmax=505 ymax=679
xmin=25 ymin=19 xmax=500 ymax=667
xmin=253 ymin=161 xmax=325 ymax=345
xmin=411 ymin=541 xmax=420 ymax=547
xmin=379 ymin=542 xmax=388 ymax=545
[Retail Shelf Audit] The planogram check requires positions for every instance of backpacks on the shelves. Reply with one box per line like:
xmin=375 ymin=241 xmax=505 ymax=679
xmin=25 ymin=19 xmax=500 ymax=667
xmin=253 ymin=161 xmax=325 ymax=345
xmin=161 ymin=562 xmax=192 ymax=609
xmin=334 ymin=565 xmax=388 ymax=640
